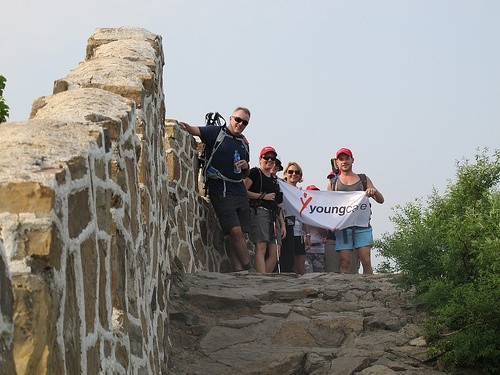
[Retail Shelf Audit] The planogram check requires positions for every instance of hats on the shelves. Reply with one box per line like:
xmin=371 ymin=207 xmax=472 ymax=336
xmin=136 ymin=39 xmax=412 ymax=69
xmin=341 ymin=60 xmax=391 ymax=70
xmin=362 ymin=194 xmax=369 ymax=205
xmin=327 ymin=170 xmax=335 ymax=179
xmin=260 ymin=146 xmax=277 ymax=157
xmin=336 ymin=148 xmax=352 ymax=158
xmin=306 ymin=185 xmax=320 ymax=191
xmin=275 ymin=158 xmax=283 ymax=171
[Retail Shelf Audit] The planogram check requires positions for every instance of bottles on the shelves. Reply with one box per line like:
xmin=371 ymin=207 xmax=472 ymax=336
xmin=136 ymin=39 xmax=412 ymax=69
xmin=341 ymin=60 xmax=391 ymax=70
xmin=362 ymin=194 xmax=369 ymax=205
xmin=233 ymin=149 xmax=241 ymax=173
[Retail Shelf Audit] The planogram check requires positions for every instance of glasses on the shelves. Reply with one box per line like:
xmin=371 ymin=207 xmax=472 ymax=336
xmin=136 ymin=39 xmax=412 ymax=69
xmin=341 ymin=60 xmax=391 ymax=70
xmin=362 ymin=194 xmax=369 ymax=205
xmin=288 ymin=170 xmax=300 ymax=174
xmin=263 ymin=155 xmax=276 ymax=161
xmin=233 ymin=115 xmax=248 ymax=126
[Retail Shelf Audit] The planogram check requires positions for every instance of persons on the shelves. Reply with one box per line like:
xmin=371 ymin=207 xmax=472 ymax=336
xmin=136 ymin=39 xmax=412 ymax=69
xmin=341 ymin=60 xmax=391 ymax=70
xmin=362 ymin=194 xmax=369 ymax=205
xmin=276 ymin=162 xmax=306 ymax=275
xmin=245 ymin=146 xmax=287 ymax=274
xmin=327 ymin=147 xmax=384 ymax=275
xmin=327 ymin=169 xmax=341 ymax=179
xmin=180 ymin=107 xmax=251 ymax=272
xmin=303 ymin=185 xmax=329 ymax=273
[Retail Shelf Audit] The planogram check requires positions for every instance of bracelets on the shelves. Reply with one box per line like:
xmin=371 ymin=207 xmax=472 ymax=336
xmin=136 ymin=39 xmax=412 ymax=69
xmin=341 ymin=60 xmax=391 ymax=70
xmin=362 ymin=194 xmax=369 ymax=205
xmin=307 ymin=233 xmax=310 ymax=236
xmin=281 ymin=222 xmax=285 ymax=224
